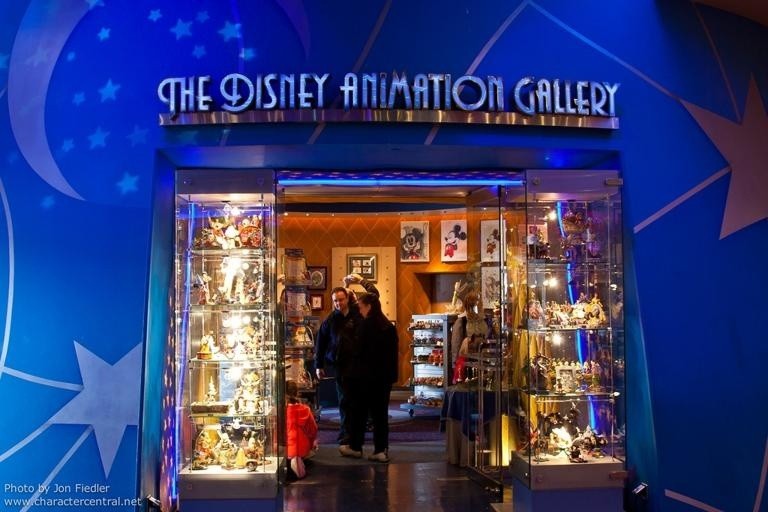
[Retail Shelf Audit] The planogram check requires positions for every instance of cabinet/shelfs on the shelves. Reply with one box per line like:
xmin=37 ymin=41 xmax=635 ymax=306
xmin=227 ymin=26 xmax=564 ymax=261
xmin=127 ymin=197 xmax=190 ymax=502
xmin=399 ymin=314 xmax=457 ymax=420
xmin=500 ymin=168 xmax=628 ymax=491
xmin=174 ymin=168 xmax=286 ymax=500
xmin=284 ymin=271 xmax=322 ymax=424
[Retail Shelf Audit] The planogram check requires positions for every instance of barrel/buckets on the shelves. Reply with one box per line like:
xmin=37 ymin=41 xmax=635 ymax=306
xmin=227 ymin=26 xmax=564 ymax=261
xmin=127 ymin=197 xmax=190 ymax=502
xmin=284 ymin=355 xmax=305 ymax=384
xmin=283 ymin=248 xmax=307 ymax=285
xmin=285 ymin=322 xmax=307 ymax=346
xmin=284 ymin=288 xmax=307 ymax=317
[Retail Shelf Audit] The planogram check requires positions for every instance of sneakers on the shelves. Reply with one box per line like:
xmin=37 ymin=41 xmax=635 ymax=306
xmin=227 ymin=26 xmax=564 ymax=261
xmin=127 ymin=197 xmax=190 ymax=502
xmin=340 ymin=445 xmax=363 ymax=458
xmin=368 ymin=452 xmax=389 ymax=462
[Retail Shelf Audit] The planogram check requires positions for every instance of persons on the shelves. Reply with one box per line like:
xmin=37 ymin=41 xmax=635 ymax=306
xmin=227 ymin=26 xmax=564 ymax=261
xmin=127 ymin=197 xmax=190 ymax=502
xmin=284 ymin=379 xmax=319 ymax=477
xmin=313 ymin=273 xmax=399 ymax=462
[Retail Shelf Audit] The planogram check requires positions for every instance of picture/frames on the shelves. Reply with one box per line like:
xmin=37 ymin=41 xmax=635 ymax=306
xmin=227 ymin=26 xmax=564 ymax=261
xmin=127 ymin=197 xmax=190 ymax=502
xmin=310 ymin=294 xmax=324 ymax=311
xmin=347 ymin=253 xmax=378 ymax=283
xmin=307 ymin=266 xmax=328 ymax=290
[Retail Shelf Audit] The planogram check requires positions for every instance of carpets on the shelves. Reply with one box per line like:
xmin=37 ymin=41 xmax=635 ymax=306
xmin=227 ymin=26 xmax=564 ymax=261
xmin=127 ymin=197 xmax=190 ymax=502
xmin=317 ymin=399 xmax=447 ymax=444
